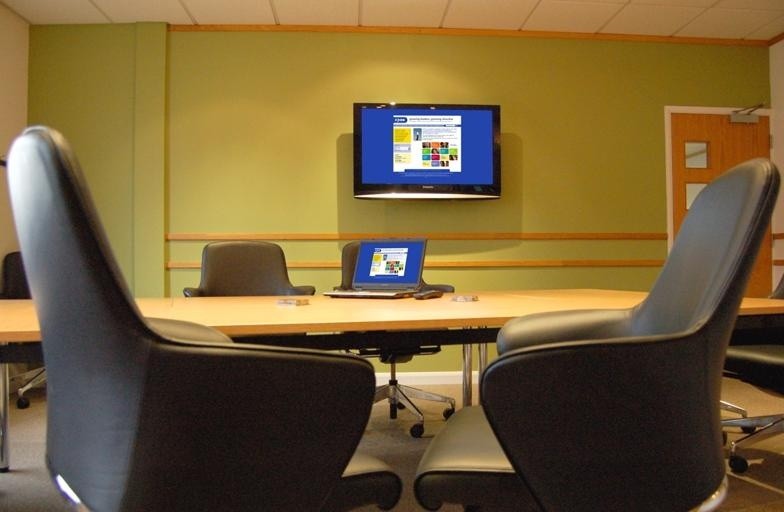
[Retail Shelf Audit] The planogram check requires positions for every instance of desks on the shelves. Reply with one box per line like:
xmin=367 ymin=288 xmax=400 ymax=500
xmin=2 ymin=287 xmax=783 ymax=473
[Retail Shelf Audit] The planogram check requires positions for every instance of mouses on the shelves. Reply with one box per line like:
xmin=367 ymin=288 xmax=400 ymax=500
xmin=412 ymin=289 xmax=442 ymax=300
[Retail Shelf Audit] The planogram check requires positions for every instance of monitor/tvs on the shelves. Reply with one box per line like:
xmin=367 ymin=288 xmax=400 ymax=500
xmin=352 ymin=102 xmax=502 ymax=201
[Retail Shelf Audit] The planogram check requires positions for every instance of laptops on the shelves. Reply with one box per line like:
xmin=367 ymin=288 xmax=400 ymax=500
xmin=322 ymin=236 xmax=429 ymax=299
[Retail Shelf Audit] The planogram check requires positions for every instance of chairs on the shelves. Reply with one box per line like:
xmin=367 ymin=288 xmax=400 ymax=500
xmin=327 ymin=237 xmax=456 ymax=437
xmin=719 ymin=276 xmax=784 ymax=477
xmin=1 ymin=248 xmax=55 ymax=406
xmin=4 ymin=124 xmax=405 ymax=511
xmin=182 ymin=241 xmax=315 ymax=296
xmin=412 ymin=156 xmax=780 ymax=512
